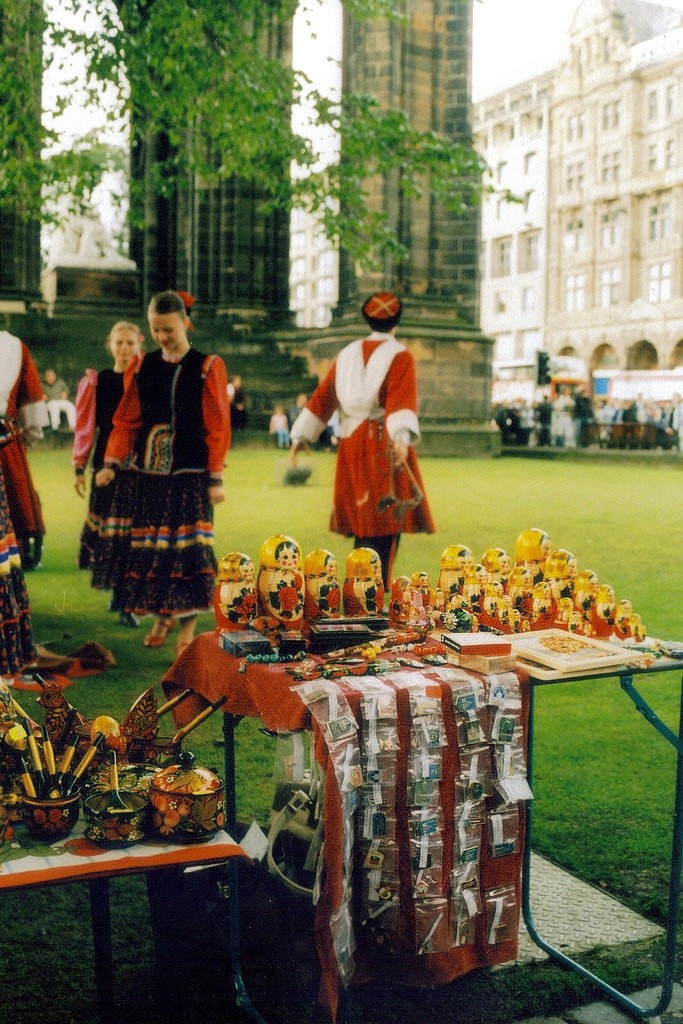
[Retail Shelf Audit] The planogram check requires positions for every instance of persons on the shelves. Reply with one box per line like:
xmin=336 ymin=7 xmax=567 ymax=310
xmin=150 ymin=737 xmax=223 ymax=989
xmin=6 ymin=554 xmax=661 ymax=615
xmin=230 ymin=375 xmax=248 ymax=434
xmin=41 ymin=369 xmax=77 ymax=431
xmin=269 ymin=391 xmax=338 ymax=454
xmin=0 ymin=330 xmax=48 ymax=675
xmin=490 ymin=386 xmax=682 ymax=450
xmin=291 ymin=293 xmax=436 ymax=590
xmin=214 ymin=536 xmax=384 ymax=630
xmin=74 ymin=321 xmax=148 ymax=626
xmin=389 ymin=528 xmax=648 ymax=643
xmin=95 ymin=293 xmax=230 ymax=654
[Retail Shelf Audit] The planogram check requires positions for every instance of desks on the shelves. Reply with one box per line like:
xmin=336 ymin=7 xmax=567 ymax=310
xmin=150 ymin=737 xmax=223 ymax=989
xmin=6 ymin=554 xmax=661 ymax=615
xmin=0 ymin=631 xmax=683 ymax=1024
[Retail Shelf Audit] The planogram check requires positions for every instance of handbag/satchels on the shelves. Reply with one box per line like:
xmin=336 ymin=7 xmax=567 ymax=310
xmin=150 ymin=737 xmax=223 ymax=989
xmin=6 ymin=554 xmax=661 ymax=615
xmin=143 ymin=730 xmax=325 ymax=1024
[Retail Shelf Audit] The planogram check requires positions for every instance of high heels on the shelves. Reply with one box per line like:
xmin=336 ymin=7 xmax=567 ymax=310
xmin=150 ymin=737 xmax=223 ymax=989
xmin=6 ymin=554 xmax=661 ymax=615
xmin=175 ymin=642 xmax=190 ymax=658
xmin=144 ymin=618 xmax=176 ymax=647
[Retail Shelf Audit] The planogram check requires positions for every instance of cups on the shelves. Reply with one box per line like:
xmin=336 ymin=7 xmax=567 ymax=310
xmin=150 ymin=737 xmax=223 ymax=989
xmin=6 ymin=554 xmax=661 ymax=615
xmin=148 ymin=738 xmax=181 ymax=767
xmin=21 ymin=784 xmax=80 ymax=839
xmin=74 ymin=723 xmax=92 ymax=761
xmin=84 ymin=792 xmax=147 ymax=842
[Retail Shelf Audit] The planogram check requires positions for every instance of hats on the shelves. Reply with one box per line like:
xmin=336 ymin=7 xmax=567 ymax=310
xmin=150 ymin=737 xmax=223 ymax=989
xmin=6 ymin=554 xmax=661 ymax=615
xmin=361 ymin=292 xmax=402 ymax=331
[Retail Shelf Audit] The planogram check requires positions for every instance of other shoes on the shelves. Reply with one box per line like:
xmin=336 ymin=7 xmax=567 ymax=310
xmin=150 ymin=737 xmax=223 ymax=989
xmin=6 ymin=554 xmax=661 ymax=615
xmin=118 ymin=608 xmax=140 ymax=627
xmin=109 ymin=601 xmax=127 ymax=611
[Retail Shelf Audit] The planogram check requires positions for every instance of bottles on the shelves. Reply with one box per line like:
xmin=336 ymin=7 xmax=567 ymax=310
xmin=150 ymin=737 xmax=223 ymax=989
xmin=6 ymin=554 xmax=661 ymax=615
xmin=150 ymin=750 xmax=226 ymax=844
